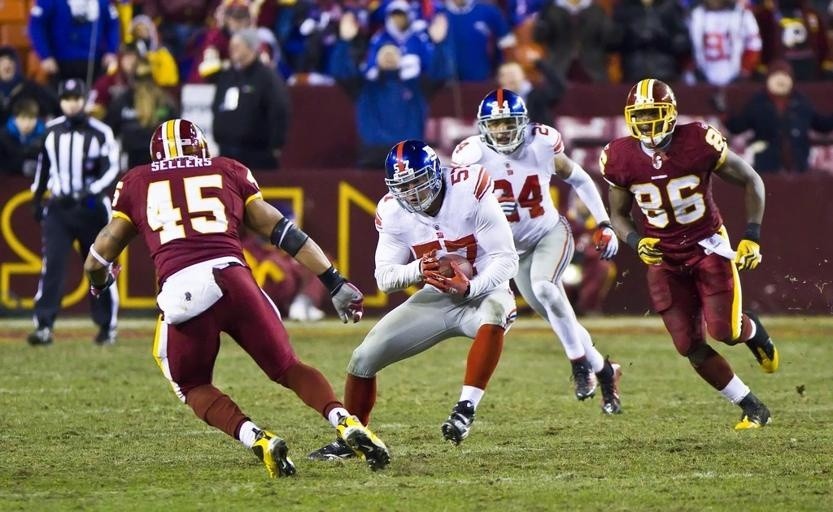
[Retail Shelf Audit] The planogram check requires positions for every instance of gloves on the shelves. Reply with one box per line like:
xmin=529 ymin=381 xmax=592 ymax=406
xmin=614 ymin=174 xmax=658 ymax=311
xmin=626 ymin=232 xmax=664 ymax=267
xmin=91 ymin=260 xmax=122 ymax=300
xmin=593 ymin=223 xmax=618 ymax=261
xmin=319 ymin=265 xmax=365 ymax=324
xmin=66 ymin=184 xmax=94 ymax=205
xmin=735 ymin=222 xmax=763 ymax=271
xmin=33 ymin=202 xmax=44 ymax=223
xmin=424 ymin=259 xmax=471 ymax=299
xmin=419 ymin=250 xmax=436 ymax=279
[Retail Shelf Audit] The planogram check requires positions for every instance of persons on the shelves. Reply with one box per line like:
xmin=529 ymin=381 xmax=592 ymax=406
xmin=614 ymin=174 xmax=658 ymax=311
xmin=308 ymin=140 xmax=519 ymax=461
xmin=1 ymin=82 xmax=49 ymax=176
xmin=191 ymin=4 xmax=271 ymax=83
xmin=82 ymin=119 xmax=390 ymax=478
xmin=369 ymin=1 xmax=436 ymax=83
xmin=495 ymin=63 xmax=557 ymax=134
xmin=122 ymin=14 xmax=177 ymax=87
xmin=615 ymin=1 xmax=692 ymax=81
xmin=723 ymin=58 xmax=833 ymax=173
xmin=763 ymin=1 xmax=828 ymax=66
xmin=26 ymin=77 xmax=124 ymax=347
xmin=104 ymin=62 xmax=180 ymax=168
xmin=591 ymin=77 xmax=777 ymax=429
xmin=274 ymin=0 xmax=322 ymax=82
xmin=29 ymin=1 xmax=120 ymax=86
xmin=533 ymin=1 xmax=622 ymax=86
xmin=686 ymin=1 xmax=762 ymax=86
xmin=353 ymin=44 xmax=426 ymax=167
xmin=325 ymin=8 xmax=368 ymax=78
xmin=1 ymin=46 xmax=61 ymax=128
xmin=429 ymin=1 xmax=512 ymax=84
xmin=202 ymin=29 xmax=290 ymax=170
xmin=448 ymin=88 xmax=624 ymax=416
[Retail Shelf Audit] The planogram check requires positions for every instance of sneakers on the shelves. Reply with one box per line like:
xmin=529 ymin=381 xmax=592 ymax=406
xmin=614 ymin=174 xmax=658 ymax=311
xmin=746 ymin=311 xmax=779 ymax=375
xmin=441 ymin=399 xmax=476 ymax=445
xmin=310 ymin=440 xmax=355 ymax=460
xmin=29 ymin=323 xmax=55 ymax=347
xmin=598 ymin=355 xmax=622 ymax=414
xmin=571 ymin=358 xmax=597 ymax=401
xmin=95 ymin=327 xmax=116 ymax=349
xmin=736 ymin=393 xmax=770 ymax=429
xmin=253 ymin=429 xmax=296 ymax=480
xmin=337 ymin=415 xmax=392 ymax=473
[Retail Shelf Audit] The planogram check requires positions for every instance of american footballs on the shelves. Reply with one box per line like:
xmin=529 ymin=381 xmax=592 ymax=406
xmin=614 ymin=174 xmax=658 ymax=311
xmin=433 ymin=255 xmax=474 ymax=281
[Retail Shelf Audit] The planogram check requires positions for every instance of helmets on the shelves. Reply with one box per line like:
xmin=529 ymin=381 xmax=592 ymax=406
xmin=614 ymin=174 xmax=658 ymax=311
xmin=150 ymin=120 xmax=208 ymax=155
xmin=626 ymin=76 xmax=675 ymax=146
xmin=477 ymin=90 xmax=531 ymax=151
xmin=385 ymin=138 xmax=444 ymax=213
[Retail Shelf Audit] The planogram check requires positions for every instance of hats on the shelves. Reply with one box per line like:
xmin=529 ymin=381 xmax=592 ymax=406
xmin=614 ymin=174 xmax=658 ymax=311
xmin=59 ymin=78 xmax=90 ymax=99
xmin=384 ymin=1 xmax=411 ymax=13
